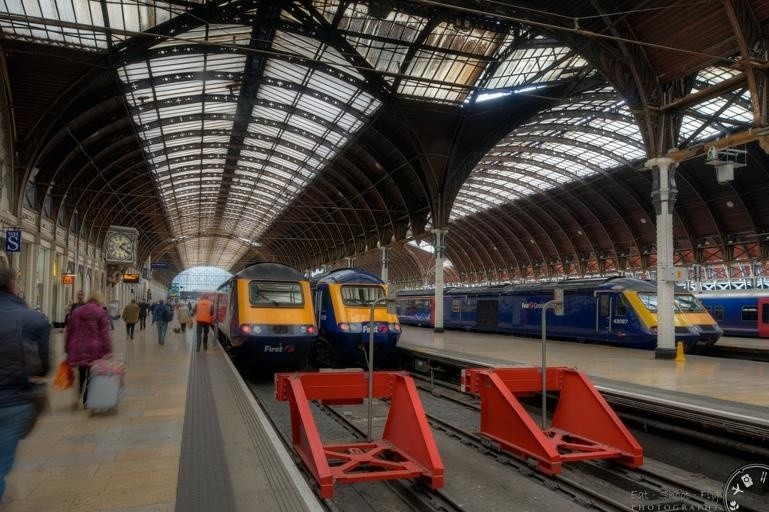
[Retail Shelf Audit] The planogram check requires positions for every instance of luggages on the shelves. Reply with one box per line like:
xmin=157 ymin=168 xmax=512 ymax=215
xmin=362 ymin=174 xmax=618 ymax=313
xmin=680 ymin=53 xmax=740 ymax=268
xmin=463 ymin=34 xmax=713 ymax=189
xmin=86 ymin=354 xmax=126 ymax=415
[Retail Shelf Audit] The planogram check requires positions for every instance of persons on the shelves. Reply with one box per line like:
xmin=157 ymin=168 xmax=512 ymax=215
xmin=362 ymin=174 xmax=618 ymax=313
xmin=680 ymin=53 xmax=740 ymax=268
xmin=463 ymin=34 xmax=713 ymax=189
xmin=62 ymin=290 xmax=216 ymax=353
xmin=0 ymin=264 xmax=52 ymax=504
xmin=64 ymin=290 xmax=114 ymax=410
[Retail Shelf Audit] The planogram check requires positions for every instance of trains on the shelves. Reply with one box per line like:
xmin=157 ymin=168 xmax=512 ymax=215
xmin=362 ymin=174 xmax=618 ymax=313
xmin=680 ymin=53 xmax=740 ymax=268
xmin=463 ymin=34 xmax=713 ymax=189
xmin=208 ymin=261 xmax=319 ymax=357
xmin=693 ymin=293 xmax=769 ymax=338
xmin=396 ymin=276 xmax=723 ymax=354
xmin=308 ymin=268 xmax=402 ymax=358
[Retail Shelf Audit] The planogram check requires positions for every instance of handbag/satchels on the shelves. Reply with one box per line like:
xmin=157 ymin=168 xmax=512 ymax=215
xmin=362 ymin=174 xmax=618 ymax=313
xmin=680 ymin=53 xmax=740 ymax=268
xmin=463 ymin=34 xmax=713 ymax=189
xmin=188 ymin=317 xmax=194 ymax=329
xmin=88 ymin=357 xmax=125 ymax=376
xmin=53 ymin=361 xmax=75 ymax=390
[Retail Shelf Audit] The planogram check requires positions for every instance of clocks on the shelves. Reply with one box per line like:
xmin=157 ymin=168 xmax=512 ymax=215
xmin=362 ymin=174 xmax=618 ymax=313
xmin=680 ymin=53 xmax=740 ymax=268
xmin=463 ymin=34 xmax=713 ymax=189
xmin=104 ymin=225 xmax=139 ymax=267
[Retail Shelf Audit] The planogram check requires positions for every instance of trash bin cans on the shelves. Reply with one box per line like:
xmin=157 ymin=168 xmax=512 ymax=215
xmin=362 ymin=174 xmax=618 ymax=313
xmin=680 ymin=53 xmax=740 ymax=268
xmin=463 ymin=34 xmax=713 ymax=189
xmin=108 ymin=303 xmax=120 ymax=320
